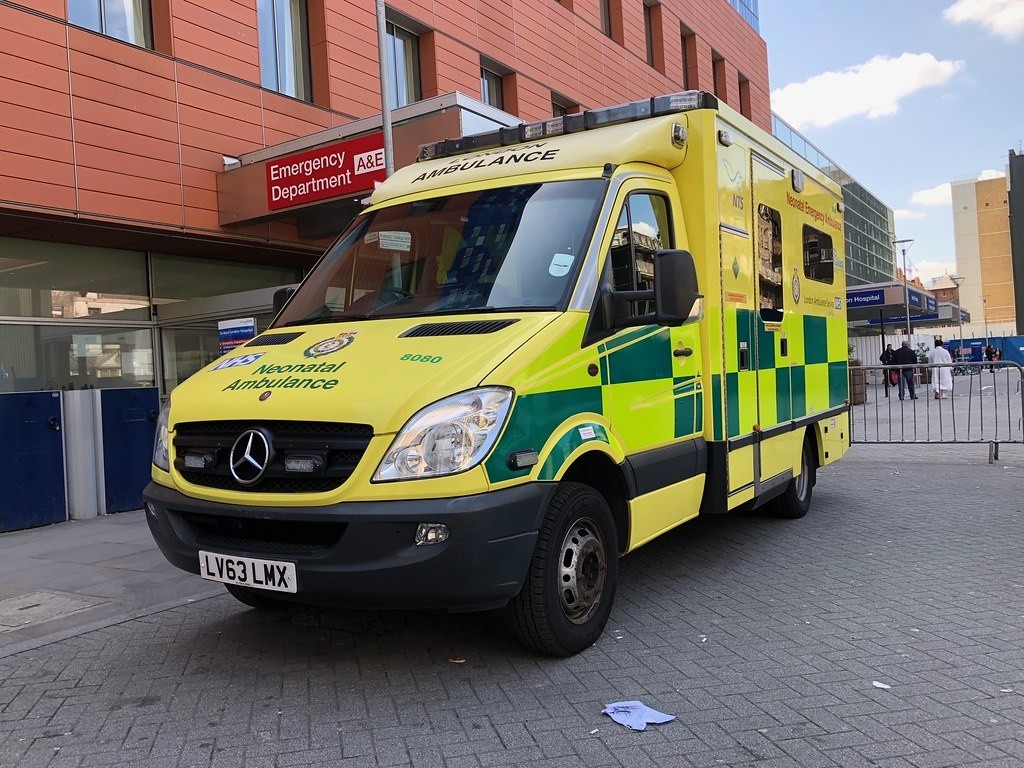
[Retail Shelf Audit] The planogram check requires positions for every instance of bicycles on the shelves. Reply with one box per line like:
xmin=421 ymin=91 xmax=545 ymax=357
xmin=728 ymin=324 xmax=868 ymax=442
xmin=950 ymin=348 xmax=980 ymax=376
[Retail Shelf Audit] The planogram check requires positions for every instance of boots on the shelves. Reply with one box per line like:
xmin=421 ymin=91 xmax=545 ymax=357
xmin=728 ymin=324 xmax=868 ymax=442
xmin=935 ymin=389 xmax=940 ymax=400
xmin=941 ymin=390 xmax=948 ymax=399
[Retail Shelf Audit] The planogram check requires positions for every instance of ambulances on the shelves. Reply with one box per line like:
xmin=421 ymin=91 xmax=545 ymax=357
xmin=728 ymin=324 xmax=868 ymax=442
xmin=143 ymin=89 xmax=856 ymax=661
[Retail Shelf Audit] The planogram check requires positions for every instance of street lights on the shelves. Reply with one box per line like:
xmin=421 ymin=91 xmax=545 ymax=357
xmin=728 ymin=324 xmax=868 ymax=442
xmin=980 ymin=295 xmax=991 ymax=347
xmin=950 ymin=276 xmax=966 ymax=362
xmin=893 ymin=239 xmax=916 ymax=351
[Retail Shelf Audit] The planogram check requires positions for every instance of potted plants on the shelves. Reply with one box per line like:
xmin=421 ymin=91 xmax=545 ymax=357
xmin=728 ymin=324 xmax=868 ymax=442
xmin=849 ymin=359 xmax=868 ymax=404
xmin=918 ymin=357 xmax=932 ymax=384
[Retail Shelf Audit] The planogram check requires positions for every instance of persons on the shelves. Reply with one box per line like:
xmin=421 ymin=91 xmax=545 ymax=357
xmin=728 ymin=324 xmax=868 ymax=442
xmin=995 ymin=348 xmax=1002 ymax=373
xmin=892 ymin=341 xmax=918 ymax=400
xmin=928 ymin=340 xmax=953 ymax=399
xmin=985 ymin=345 xmax=995 ymax=373
xmin=879 ymin=344 xmax=896 ymax=387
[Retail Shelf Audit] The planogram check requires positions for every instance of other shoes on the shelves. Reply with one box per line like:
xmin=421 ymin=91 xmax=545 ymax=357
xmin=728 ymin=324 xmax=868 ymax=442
xmin=911 ymin=394 xmax=918 ymax=399
